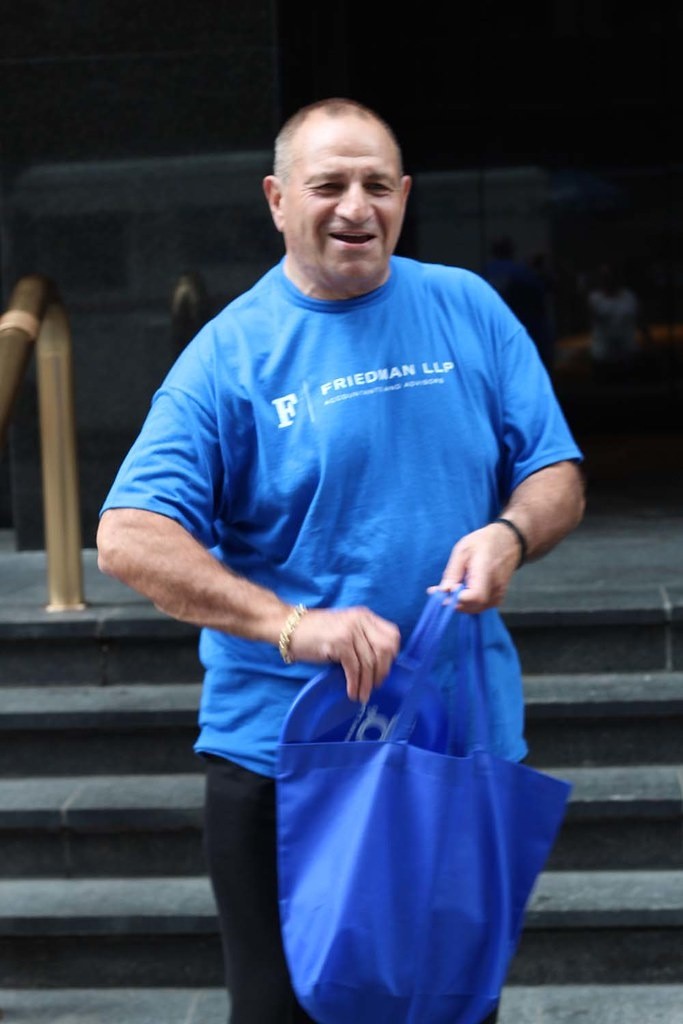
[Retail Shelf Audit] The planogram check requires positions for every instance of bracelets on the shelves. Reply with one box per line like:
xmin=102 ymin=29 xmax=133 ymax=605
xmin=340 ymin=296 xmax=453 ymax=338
xmin=279 ymin=603 xmax=309 ymax=664
xmin=490 ymin=517 xmax=528 ymax=570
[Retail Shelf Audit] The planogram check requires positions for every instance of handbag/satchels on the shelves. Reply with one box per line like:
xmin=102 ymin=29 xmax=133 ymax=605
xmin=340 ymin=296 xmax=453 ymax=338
xmin=275 ymin=582 xmax=571 ymax=1024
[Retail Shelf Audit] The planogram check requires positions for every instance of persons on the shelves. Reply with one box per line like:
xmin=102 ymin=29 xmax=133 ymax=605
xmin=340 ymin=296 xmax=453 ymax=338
xmin=98 ymin=102 xmax=584 ymax=1024
